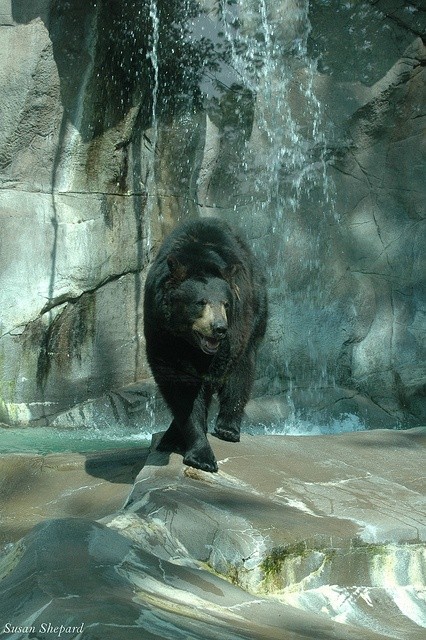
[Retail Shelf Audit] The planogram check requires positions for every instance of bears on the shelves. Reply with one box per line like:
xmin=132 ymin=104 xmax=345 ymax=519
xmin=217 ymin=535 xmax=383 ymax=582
xmin=143 ymin=218 xmax=268 ymax=471
